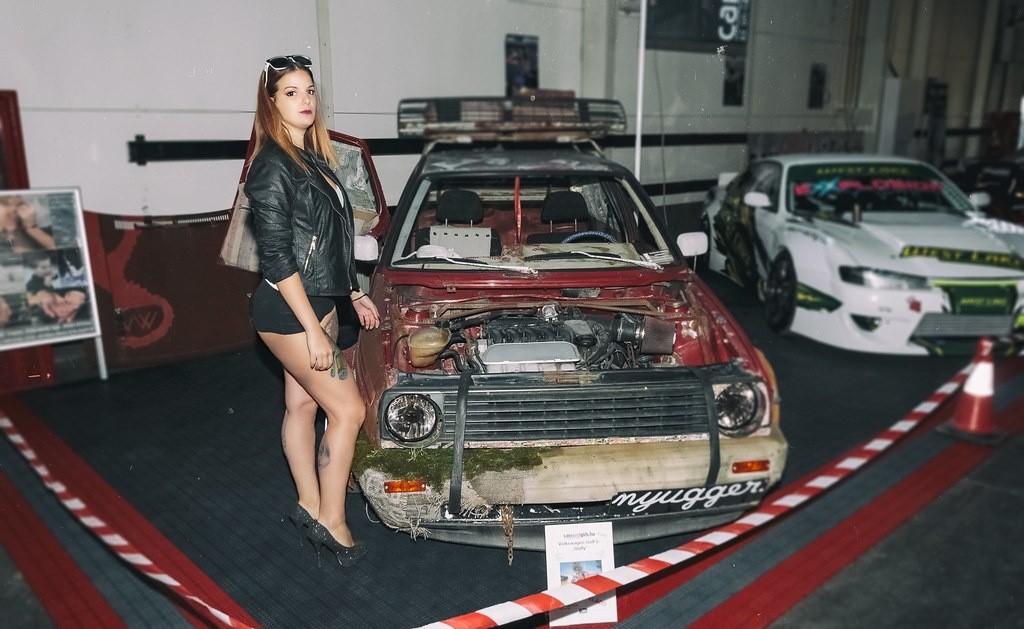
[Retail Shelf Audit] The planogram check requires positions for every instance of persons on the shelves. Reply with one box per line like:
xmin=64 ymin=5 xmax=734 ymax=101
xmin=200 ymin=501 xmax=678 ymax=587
xmin=0 ymin=194 xmax=89 ymax=327
xmin=242 ymin=57 xmax=380 ymax=567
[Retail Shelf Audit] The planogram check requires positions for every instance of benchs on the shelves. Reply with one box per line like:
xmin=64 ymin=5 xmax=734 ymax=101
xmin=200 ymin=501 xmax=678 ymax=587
xmin=416 ymin=204 xmax=590 ymax=247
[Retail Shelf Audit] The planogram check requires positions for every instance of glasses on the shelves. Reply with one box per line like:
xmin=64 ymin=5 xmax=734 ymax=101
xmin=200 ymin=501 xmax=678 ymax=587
xmin=263 ymin=54 xmax=312 ymax=88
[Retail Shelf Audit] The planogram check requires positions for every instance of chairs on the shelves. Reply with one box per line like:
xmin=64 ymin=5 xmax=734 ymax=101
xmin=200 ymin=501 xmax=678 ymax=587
xmin=414 ymin=190 xmax=503 ymax=259
xmin=527 ymin=190 xmax=607 ymax=247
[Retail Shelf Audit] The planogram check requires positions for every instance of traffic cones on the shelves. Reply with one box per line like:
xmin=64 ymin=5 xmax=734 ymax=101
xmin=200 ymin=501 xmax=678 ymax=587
xmin=937 ymin=340 xmax=1012 ymax=449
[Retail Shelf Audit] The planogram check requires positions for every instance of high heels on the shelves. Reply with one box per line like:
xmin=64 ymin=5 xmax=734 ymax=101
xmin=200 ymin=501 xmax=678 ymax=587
xmin=289 ymin=503 xmax=316 ymax=547
xmin=308 ymin=518 xmax=368 ymax=569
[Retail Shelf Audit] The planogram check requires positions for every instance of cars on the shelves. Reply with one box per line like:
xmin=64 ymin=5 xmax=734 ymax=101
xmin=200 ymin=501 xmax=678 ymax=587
xmin=700 ymin=154 xmax=1024 ymax=358
xmin=352 ymin=136 xmax=786 ymax=550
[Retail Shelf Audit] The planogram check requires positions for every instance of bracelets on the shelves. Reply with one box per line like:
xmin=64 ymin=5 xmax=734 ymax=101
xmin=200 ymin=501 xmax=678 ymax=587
xmin=351 ymin=294 xmax=368 ymax=302
xmin=24 ymin=224 xmax=37 ymax=229
